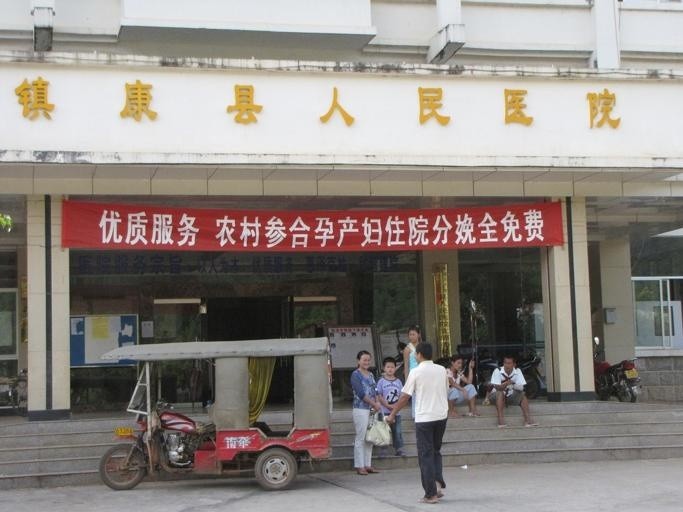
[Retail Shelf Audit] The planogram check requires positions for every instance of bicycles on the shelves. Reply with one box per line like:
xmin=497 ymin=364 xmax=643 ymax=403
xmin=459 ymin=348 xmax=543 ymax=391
xmin=10 ymin=368 xmax=28 ymax=416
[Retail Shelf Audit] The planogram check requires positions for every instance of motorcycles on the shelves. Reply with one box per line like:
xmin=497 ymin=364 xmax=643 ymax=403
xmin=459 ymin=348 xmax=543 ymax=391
xmin=389 ymin=341 xmax=471 ymax=390
xmin=478 ymin=354 xmax=545 ymax=399
xmin=593 ymin=337 xmax=642 ymax=403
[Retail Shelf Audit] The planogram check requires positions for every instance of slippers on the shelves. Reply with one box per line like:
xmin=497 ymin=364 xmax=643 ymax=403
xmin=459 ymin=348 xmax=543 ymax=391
xmin=436 ymin=490 xmax=443 ymax=498
xmin=418 ymin=496 xmax=439 ymax=504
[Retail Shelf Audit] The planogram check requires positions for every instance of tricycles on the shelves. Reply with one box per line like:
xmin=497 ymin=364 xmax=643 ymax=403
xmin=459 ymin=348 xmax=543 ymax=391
xmin=99 ymin=336 xmax=332 ymax=491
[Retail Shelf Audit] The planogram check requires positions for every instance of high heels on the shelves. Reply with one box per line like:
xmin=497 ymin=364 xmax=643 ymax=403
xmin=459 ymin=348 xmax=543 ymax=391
xmin=356 ymin=467 xmax=368 ymax=476
xmin=365 ymin=466 xmax=380 ymax=473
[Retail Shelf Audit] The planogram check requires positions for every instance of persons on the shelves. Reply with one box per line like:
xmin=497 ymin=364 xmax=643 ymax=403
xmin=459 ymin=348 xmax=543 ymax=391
xmin=403 ymin=325 xmax=423 ymax=420
xmin=377 ymin=357 xmax=407 ymax=459
xmin=446 ymin=356 xmax=482 ymax=417
xmin=350 ymin=350 xmax=382 ymax=475
xmin=489 ymin=353 xmax=540 ymax=428
xmin=385 ymin=341 xmax=449 ymax=504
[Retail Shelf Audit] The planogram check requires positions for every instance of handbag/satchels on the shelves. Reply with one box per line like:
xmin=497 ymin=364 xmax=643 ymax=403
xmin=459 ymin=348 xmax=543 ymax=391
xmin=364 ymin=419 xmax=394 ymax=448
